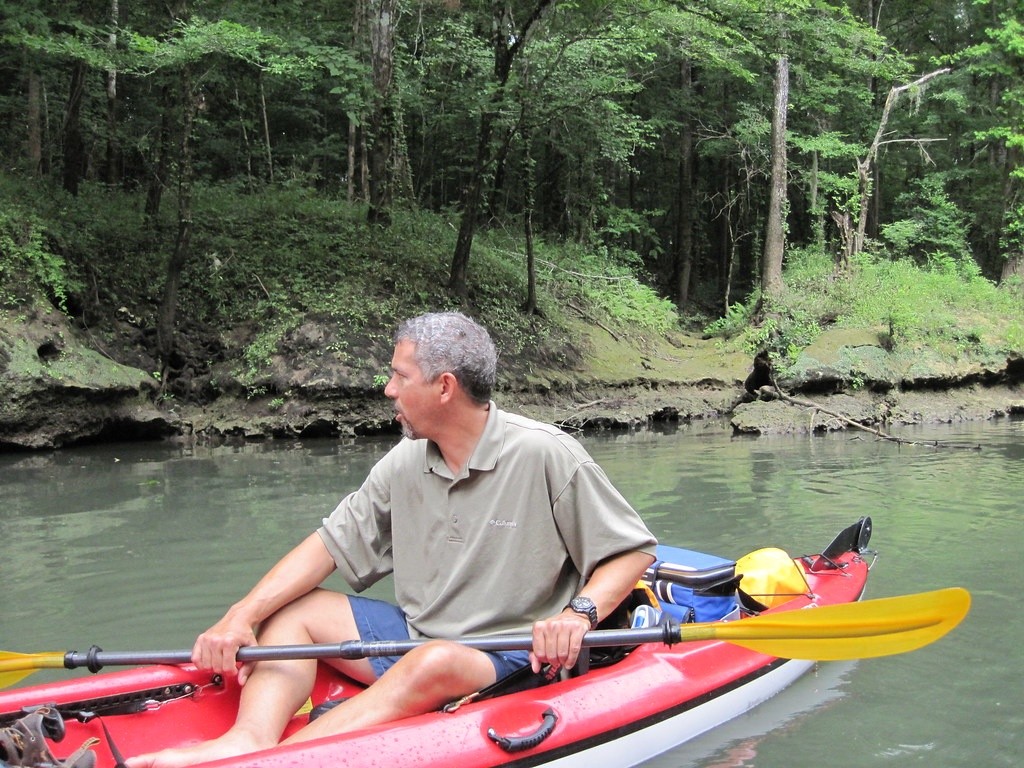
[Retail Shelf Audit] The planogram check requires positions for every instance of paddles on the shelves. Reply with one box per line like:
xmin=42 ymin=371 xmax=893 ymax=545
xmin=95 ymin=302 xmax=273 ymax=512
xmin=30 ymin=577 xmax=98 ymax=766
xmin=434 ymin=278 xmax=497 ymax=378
xmin=0 ymin=586 xmax=969 ymax=695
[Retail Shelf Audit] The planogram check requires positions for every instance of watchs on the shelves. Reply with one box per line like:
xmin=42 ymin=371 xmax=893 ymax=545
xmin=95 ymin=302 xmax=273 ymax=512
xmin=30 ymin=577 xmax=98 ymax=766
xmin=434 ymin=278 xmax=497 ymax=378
xmin=562 ymin=596 xmax=598 ymax=631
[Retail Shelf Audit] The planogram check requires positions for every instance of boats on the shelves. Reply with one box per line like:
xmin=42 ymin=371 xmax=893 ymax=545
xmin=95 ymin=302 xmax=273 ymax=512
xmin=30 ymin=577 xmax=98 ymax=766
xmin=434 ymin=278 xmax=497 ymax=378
xmin=0 ymin=514 xmax=879 ymax=766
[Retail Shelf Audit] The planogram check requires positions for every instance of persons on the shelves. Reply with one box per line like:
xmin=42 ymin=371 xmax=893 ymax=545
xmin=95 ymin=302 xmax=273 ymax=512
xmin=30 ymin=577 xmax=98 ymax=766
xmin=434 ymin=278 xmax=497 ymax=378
xmin=125 ymin=312 xmax=657 ymax=766
